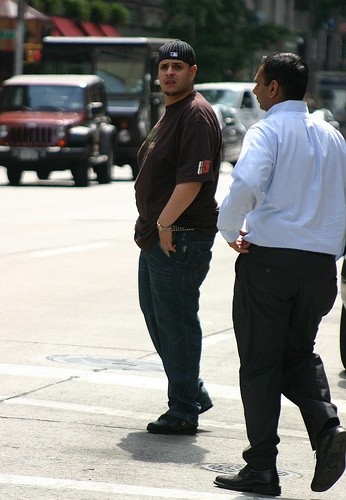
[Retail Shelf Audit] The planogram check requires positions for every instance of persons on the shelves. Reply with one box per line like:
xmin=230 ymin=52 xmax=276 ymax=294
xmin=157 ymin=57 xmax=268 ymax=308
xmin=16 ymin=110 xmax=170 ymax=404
xmin=133 ymin=40 xmax=223 ymax=434
xmin=213 ymin=53 xmax=346 ymax=496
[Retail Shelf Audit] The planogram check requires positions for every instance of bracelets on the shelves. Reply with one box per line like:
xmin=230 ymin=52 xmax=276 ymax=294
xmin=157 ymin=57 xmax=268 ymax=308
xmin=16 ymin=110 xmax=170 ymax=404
xmin=156 ymin=220 xmax=171 ymax=231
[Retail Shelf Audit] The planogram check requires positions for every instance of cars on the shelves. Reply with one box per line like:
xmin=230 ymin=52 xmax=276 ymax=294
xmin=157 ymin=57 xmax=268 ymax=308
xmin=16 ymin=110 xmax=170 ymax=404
xmin=308 ymin=69 xmax=346 ymax=138
xmin=194 ymin=81 xmax=267 ymax=168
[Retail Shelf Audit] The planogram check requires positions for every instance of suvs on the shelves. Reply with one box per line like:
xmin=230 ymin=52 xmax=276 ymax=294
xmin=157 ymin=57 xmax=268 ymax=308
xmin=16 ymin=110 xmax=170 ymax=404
xmin=0 ymin=74 xmax=122 ymax=187
xmin=30 ymin=35 xmax=174 ymax=180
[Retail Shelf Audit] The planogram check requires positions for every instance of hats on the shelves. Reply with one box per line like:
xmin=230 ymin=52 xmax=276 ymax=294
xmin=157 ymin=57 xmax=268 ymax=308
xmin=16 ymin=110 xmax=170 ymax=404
xmin=158 ymin=41 xmax=197 ymax=67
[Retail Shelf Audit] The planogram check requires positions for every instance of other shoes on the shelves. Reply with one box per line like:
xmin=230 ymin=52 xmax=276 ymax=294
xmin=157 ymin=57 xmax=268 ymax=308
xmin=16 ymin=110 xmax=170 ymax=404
xmin=310 ymin=425 xmax=346 ymax=493
xmin=198 ymin=392 xmax=214 ymax=413
xmin=147 ymin=414 xmax=198 ymax=435
xmin=214 ymin=465 xmax=282 ymax=496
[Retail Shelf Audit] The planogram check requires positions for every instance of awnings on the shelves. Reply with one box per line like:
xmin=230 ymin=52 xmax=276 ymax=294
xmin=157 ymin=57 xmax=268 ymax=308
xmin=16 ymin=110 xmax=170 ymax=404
xmin=51 ymin=17 xmax=120 ymax=37
